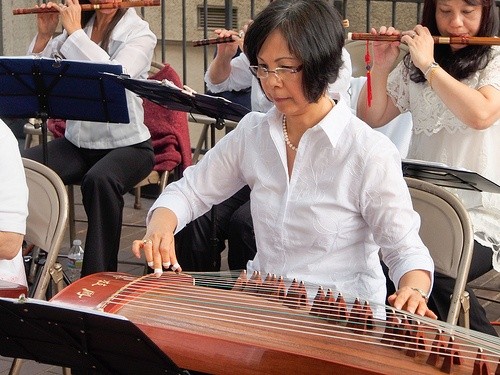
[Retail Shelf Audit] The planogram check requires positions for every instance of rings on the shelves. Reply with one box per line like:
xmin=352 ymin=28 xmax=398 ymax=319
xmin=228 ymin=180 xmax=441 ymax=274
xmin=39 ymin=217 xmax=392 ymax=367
xmin=239 ymin=32 xmax=243 ymax=37
xmin=63 ymin=6 xmax=67 ymax=9
xmin=142 ymin=239 xmax=153 ymax=247
xmin=412 ymin=33 xmax=416 ymax=38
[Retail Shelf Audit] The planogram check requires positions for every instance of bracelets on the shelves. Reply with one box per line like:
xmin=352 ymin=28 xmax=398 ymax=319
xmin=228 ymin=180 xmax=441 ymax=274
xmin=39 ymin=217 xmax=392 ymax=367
xmin=430 ymin=69 xmax=438 ymax=90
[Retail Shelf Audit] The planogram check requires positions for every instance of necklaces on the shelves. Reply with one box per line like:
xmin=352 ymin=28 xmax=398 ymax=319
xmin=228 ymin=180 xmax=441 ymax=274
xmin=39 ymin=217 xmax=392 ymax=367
xmin=282 ymin=96 xmax=336 ymax=152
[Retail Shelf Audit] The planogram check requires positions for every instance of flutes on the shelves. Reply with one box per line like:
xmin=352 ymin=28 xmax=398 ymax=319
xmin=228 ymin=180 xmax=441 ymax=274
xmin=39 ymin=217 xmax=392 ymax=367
xmin=13 ymin=0 xmax=160 ymax=16
xmin=348 ymin=31 xmax=500 ymax=47
xmin=192 ymin=37 xmax=235 ymax=47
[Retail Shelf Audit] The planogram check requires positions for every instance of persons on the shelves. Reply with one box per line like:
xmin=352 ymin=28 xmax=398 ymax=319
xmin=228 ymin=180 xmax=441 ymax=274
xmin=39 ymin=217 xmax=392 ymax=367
xmin=0 ymin=121 xmax=29 ymax=303
xmin=356 ymin=0 xmax=500 ymax=337
xmin=19 ymin=0 xmax=157 ymax=280
xmin=133 ymin=0 xmax=438 ymax=321
xmin=145 ymin=0 xmax=352 ymax=278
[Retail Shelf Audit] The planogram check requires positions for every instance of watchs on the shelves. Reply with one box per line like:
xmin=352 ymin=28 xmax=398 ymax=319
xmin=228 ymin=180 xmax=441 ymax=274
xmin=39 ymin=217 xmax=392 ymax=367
xmin=423 ymin=62 xmax=439 ymax=75
xmin=411 ymin=287 xmax=428 ymax=302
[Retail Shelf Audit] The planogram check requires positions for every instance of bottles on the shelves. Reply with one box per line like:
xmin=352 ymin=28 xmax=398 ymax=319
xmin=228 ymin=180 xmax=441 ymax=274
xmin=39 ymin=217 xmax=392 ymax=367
xmin=67 ymin=239 xmax=84 ymax=282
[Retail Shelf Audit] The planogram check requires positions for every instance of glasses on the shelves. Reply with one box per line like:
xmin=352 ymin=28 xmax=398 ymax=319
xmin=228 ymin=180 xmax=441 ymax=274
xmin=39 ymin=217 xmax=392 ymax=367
xmin=248 ymin=60 xmax=304 ymax=80
